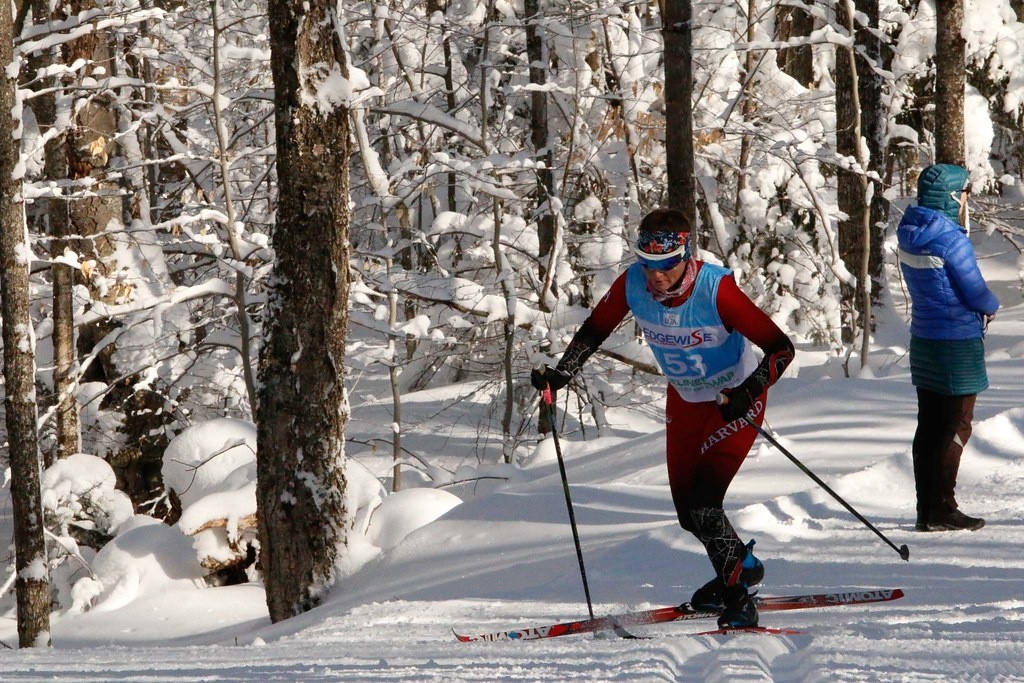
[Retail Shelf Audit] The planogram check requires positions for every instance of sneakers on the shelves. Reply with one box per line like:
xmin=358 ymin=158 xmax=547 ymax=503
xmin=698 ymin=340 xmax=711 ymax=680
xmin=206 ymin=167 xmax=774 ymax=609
xmin=916 ymin=508 xmax=986 ymax=533
xmin=715 ymin=581 xmax=759 ymax=632
xmin=690 ymin=538 xmax=765 ymax=614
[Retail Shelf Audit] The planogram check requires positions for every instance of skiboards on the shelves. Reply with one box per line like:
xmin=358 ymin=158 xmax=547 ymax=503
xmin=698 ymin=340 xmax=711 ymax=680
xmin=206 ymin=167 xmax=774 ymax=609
xmin=452 ymin=586 xmax=907 ymax=641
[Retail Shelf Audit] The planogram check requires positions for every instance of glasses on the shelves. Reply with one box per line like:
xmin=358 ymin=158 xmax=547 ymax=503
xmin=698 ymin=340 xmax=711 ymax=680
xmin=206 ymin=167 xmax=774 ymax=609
xmin=633 ymin=241 xmax=686 ymax=272
xmin=956 ymin=183 xmax=972 ymax=195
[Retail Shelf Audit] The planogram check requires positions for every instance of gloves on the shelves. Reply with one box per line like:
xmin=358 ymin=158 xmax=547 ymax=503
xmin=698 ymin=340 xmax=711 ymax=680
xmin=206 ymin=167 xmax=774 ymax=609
xmin=720 ymin=377 xmax=765 ymax=421
xmin=530 ymin=364 xmax=569 ymax=391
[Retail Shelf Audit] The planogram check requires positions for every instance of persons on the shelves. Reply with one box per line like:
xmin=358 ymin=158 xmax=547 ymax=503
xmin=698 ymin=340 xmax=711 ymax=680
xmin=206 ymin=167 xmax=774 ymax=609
xmin=897 ymin=163 xmax=1000 ymax=533
xmin=530 ymin=209 xmax=795 ymax=628
